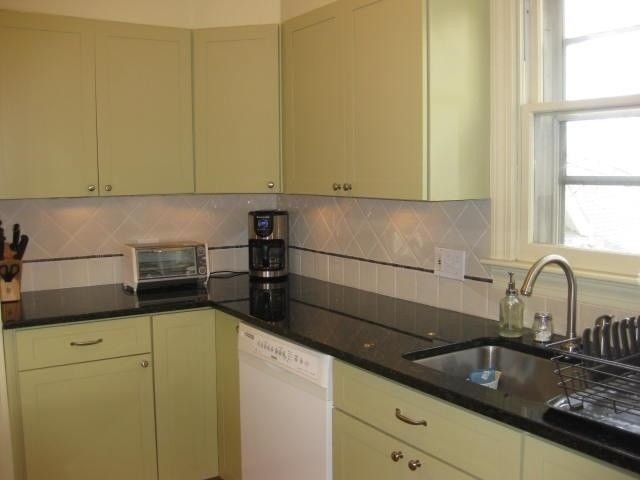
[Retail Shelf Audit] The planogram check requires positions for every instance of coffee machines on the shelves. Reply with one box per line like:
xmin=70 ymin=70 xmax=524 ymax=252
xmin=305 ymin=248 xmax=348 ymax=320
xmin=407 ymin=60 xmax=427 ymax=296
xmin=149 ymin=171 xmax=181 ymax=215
xmin=248 ymin=210 xmax=290 ymax=280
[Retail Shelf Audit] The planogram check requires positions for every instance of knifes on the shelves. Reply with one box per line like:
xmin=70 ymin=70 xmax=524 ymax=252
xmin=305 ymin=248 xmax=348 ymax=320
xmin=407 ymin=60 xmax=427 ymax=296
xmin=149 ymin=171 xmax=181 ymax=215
xmin=0 ymin=222 xmax=29 ymax=260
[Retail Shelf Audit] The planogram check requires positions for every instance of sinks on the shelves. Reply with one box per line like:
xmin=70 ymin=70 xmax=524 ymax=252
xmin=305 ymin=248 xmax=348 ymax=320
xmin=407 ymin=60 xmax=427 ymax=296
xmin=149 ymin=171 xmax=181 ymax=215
xmin=401 ymin=335 xmax=609 ymax=403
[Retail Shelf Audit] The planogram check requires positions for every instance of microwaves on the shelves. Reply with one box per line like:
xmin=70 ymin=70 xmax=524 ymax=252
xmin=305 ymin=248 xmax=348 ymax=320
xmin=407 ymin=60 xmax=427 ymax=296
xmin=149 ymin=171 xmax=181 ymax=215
xmin=122 ymin=240 xmax=210 ymax=296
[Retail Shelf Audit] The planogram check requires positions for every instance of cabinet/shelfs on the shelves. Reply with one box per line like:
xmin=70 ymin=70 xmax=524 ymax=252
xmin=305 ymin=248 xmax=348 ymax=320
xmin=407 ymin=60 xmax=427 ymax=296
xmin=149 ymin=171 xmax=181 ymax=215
xmin=2 ymin=308 xmax=240 ymax=480
xmin=0 ymin=0 xmax=491 ymax=201
xmin=330 ymin=359 xmax=640 ymax=480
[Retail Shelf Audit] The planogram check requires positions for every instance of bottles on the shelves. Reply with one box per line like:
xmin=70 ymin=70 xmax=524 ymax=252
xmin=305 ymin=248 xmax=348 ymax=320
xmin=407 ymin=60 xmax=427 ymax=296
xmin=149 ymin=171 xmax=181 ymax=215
xmin=531 ymin=312 xmax=552 ymax=343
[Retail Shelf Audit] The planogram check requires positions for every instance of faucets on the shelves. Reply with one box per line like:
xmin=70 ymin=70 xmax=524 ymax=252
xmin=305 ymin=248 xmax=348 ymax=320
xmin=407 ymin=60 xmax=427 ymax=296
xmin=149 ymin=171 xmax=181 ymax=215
xmin=523 ymin=251 xmax=583 ymax=354
